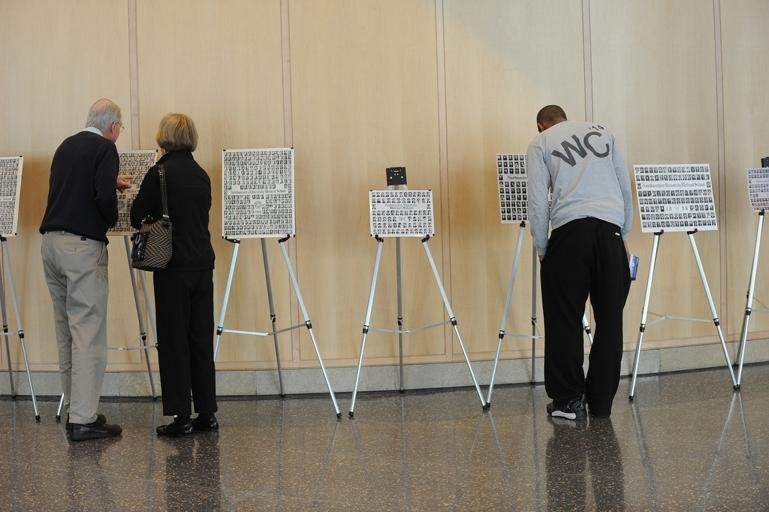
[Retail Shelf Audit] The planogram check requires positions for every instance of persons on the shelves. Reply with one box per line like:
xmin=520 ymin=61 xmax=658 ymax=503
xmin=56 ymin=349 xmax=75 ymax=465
xmin=38 ymin=98 xmax=133 ymax=444
xmin=129 ymin=112 xmax=220 ymax=438
xmin=526 ymin=105 xmax=634 ymax=421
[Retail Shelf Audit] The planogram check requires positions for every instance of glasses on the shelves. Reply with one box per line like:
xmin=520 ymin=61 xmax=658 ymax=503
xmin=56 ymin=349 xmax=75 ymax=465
xmin=118 ymin=122 xmax=126 ymax=133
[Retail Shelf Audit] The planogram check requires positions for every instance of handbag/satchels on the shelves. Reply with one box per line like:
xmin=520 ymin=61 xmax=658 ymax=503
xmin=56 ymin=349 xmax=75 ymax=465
xmin=131 ymin=215 xmax=173 ymax=272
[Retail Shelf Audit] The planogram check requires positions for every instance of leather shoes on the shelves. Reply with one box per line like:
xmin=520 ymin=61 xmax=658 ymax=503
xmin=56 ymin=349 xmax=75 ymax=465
xmin=156 ymin=421 xmax=194 ymax=438
xmin=193 ymin=418 xmax=219 ymax=431
xmin=66 ymin=413 xmax=122 ymax=441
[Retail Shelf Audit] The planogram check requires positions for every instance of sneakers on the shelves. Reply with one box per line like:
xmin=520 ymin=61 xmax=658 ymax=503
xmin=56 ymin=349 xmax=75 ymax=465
xmin=546 ymin=401 xmax=584 ymax=420
